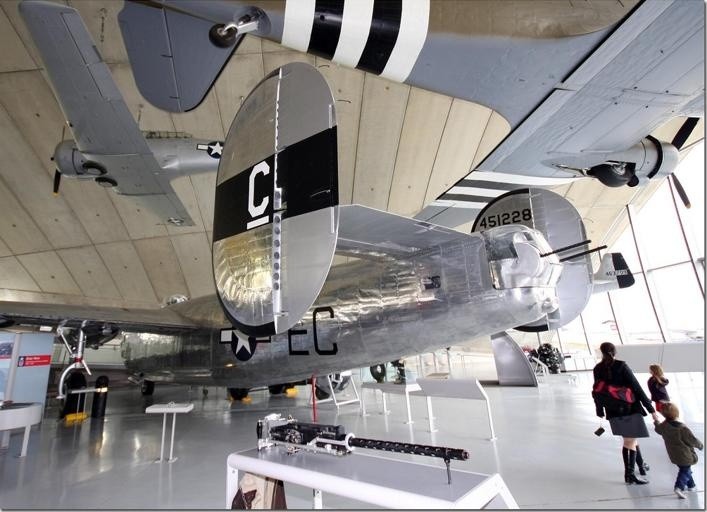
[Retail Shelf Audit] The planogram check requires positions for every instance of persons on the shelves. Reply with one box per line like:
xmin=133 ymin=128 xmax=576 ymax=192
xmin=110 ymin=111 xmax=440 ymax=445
xmin=653 ymin=402 xmax=703 ymax=499
xmin=647 ymin=364 xmax=670 ymax=413
xmin=591 ymin=342 xmax=657 ymax=485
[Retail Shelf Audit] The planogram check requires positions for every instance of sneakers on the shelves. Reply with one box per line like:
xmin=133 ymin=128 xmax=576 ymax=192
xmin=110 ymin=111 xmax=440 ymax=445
xmin=674 ymin=487 xmax=687 ymax=499
xmin=688 ymin=485 xmax=697 ymax=491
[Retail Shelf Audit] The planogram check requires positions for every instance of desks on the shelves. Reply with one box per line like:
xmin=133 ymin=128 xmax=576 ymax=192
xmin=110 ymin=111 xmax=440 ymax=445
xmin=68 ymin=387 xmax=98 ymax=424
xmin=145 ymin=402 xmax=194 ymax=463
xmin=361 ymin=382 xmax=435 ymax=424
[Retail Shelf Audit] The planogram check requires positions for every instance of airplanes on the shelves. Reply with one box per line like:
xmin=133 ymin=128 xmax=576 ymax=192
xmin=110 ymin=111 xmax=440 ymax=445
xmin=0 ymin=202 xmax=638 ymax=422
xmin=14 ymin=0 xmax=227 ymax=230
xmin=115 ymin=0 xmax=706 ymax=231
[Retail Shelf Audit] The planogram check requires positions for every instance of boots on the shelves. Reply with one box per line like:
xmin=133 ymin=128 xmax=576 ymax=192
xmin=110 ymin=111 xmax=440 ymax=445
xmin=623 ymin=446 xmax=648 ymax=484
xmin=636 ymin=445 xmax=649 ymax=475
